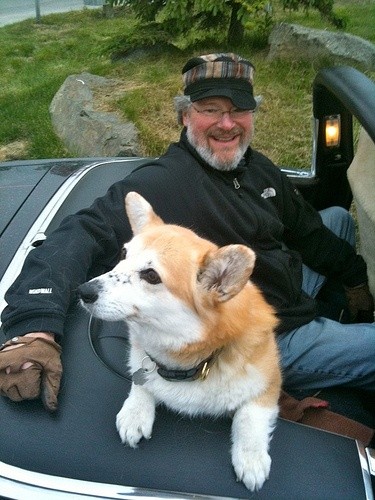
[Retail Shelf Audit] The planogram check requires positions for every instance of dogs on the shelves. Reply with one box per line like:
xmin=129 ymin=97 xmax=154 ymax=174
xmin=76 ymin=192 xmax=283 ymax=491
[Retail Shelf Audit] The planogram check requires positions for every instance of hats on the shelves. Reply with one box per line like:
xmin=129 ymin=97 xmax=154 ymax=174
xmin=182 ymin=53 xmax=257 ymax=110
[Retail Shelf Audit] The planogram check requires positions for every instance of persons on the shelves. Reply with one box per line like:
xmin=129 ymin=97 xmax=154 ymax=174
xmin=0 ymin=53 xmax=375 ymax=412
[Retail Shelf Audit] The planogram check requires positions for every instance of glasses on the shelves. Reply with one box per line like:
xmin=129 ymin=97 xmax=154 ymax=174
xmin=183 ymin=97 xmax=256 ymax=116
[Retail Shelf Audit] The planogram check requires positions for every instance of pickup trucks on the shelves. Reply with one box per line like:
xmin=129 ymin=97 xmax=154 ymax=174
xmin=0 ymin=65 xmax=375 ymax=500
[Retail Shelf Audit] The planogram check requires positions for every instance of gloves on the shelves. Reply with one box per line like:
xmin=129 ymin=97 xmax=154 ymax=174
xmin=342 ymin=283 xmax=375 ymax=323
xmin=0 ymin=336 xmax=63 ymax=414
